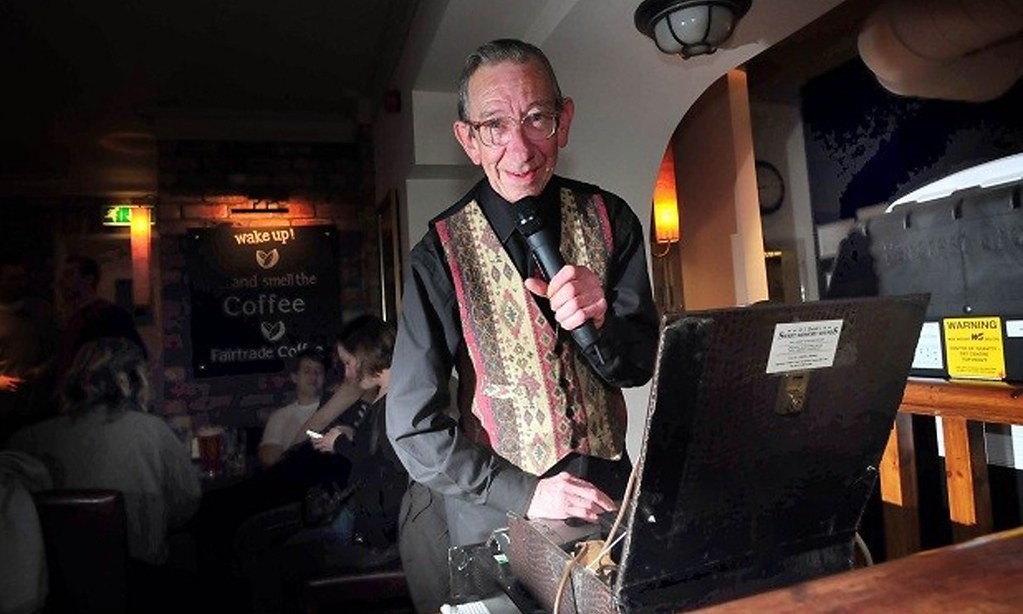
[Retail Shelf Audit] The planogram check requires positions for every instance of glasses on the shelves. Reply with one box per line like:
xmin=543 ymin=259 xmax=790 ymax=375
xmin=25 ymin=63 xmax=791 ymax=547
xmin=463 ymin=112 xmax=561 ymax=148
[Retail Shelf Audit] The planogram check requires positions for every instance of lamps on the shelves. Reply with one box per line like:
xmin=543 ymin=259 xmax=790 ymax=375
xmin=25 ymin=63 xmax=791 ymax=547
xmin=633 ymin=0 xmax=754 ymax=60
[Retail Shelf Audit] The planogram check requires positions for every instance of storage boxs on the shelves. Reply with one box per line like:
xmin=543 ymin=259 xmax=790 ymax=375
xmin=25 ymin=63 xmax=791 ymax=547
xmin=508 ymin=292 xmax=926 ymax=614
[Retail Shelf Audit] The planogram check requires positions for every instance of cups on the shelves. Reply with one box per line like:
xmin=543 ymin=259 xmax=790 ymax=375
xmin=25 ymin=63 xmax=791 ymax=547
xmin=197 ymin=431 xmax=225 ymax=467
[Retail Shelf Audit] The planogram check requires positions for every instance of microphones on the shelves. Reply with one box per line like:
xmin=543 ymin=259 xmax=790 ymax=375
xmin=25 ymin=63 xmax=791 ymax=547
xmin=509 ymin=196 xmax=600 ymax=353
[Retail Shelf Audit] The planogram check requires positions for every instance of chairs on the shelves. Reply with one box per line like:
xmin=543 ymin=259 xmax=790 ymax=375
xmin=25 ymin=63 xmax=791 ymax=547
xmin=31 ymin=489 xmax=128 ymax=584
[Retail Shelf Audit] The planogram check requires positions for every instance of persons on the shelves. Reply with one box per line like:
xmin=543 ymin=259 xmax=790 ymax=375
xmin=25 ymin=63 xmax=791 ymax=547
xmin=0 ymin=257 xmax=200 ymax=564
xmin=228 ymin=314 xmax=415 ymax=614
xmin=386 ymin=39 xmax=659 ymax=614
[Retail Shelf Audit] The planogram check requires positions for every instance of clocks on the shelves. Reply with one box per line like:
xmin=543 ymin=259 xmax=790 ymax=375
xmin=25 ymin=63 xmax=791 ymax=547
xmin=755 ymin=161 xmax=784 ymax=213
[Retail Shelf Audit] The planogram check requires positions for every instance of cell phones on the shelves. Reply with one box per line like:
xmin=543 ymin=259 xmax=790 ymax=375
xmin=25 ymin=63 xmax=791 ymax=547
xmin=306 ymin=430 xmax=323 ymax=439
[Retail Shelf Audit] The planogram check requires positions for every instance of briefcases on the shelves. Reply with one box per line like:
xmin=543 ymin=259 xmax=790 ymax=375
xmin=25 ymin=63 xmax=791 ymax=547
xmin=504 ymin=292 xmax=936 ymax=614
